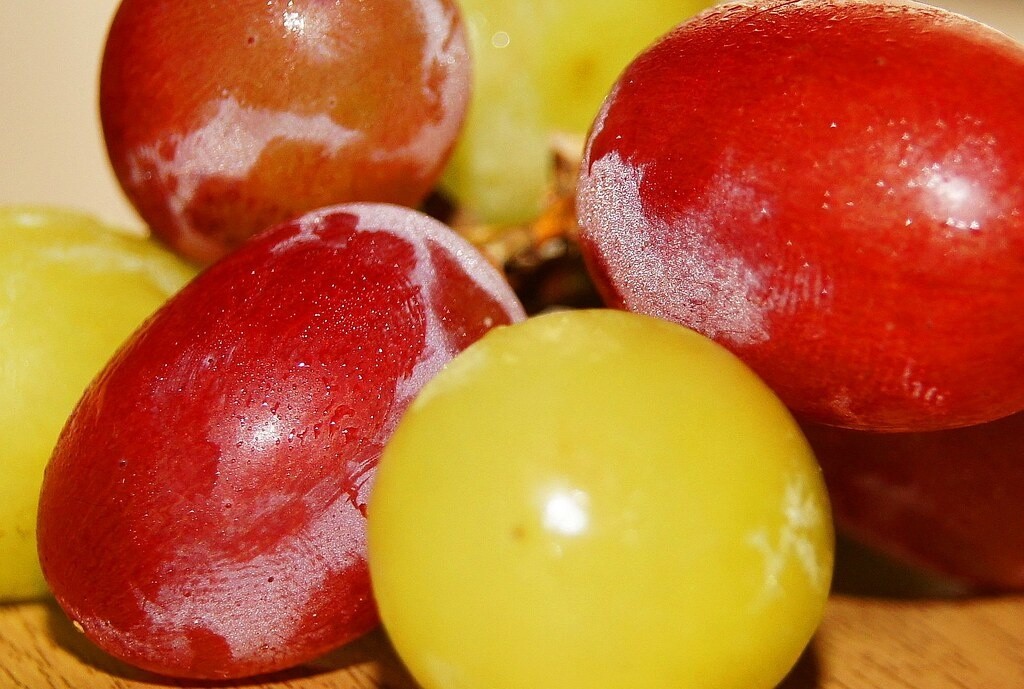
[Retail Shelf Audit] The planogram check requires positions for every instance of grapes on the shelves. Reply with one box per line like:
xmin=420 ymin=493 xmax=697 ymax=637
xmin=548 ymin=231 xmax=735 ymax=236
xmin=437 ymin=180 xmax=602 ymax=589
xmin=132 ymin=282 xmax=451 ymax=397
xmin=0 ymin=1 xmax=1024 ymax=689
